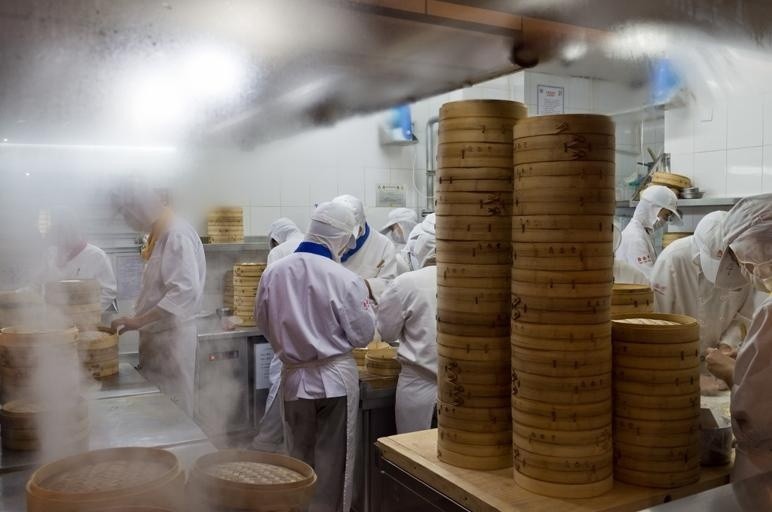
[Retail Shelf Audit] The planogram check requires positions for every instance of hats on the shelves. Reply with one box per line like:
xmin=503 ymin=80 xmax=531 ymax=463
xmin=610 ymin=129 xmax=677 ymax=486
xmin=263 ymin=217 xmax=295 ymax=248
xmin=694 ymin=210 xmax=730 ymax=283
xmin=407 ymin=212 xmax=435 ymax=266
xmin=332 ymin=196 xmax=366 ymax=237
xmin=311 ymin=200 xmax=360 ymax=251
xmin=714 ymin=194 xmax=772 ymax=292
xmin=377 ymin=206 xmax=417 ymax=234
xmin=639 ymin=183 xmax=683 ymax=228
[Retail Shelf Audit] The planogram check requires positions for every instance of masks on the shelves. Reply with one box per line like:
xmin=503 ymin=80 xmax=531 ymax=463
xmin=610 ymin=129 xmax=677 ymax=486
xmin=738 ymin=264 xmax=771 ymax=295
xmin=650 ymin=218 xmax=664 ymax=232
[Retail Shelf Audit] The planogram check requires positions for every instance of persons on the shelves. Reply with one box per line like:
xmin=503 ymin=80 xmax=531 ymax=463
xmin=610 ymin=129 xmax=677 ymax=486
xmin=609 ymin=184 xmax=772 ymax=512
xmin=250 ymin=195 xmax=438 ymax=512
xmin=54 ymin=172 xmax=206 ymax=415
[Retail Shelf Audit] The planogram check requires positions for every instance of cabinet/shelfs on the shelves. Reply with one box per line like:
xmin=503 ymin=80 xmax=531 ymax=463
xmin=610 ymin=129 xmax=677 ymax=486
xmin=370 ymin=412 xmax=738 ymax=512
xmin=351 ymin=383 xmax=400 ymax=508
xmin=198 ymin=335 xmax=273 ymax=438
